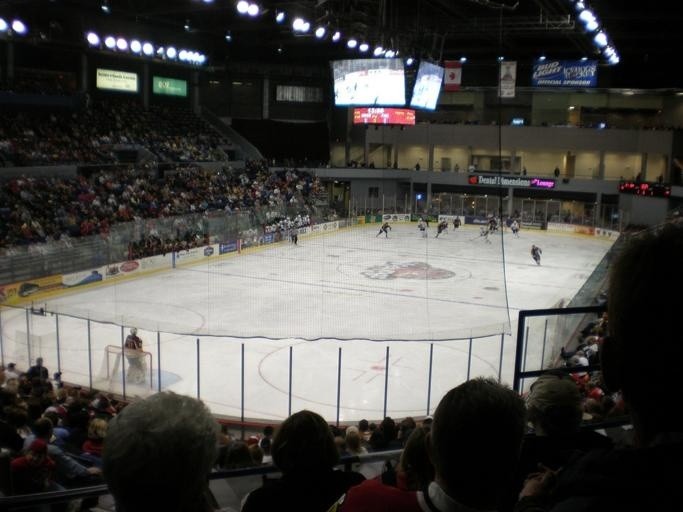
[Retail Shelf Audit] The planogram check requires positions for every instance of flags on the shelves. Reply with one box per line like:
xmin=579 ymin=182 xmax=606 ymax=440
xmin=443 ymin=61 xmax=463 ymax=93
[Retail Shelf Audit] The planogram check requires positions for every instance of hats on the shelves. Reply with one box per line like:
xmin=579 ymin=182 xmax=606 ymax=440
xmin=400 ymin=426 xmax=433 ymax=488
xmin=271 ymin=410 xmax=340 ymax=471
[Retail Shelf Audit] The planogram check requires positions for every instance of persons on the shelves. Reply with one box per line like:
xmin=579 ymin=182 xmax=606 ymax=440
xmin=122 ymin=325 xmax=143 ymax=384
xmin=529 ymin=244 xmax=542 ymax=266
xmin=554 ymin=205 xmax=682 ymax=511
